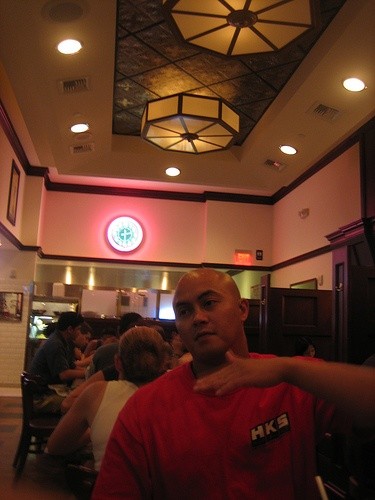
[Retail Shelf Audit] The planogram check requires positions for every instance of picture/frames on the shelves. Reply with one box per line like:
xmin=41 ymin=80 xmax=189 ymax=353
xmin=1 ymin=292 xmax=24 ymax=322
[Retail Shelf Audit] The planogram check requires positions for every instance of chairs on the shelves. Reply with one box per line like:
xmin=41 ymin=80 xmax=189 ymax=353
xmin=13 ymin=371 xmax=58 ymax=481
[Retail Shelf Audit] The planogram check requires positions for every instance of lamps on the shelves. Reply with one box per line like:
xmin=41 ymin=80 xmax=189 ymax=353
xmin=139 ymin=94 xmax=240 ymax=154
xmin=162 ymin=1 xmax=310 ymax=60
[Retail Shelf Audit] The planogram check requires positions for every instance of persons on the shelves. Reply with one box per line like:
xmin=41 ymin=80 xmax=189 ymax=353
xmin=22 ymin=310 xmax=174 ymax=473
xmin=87 ymin=264 xmax=375 ymax=500
